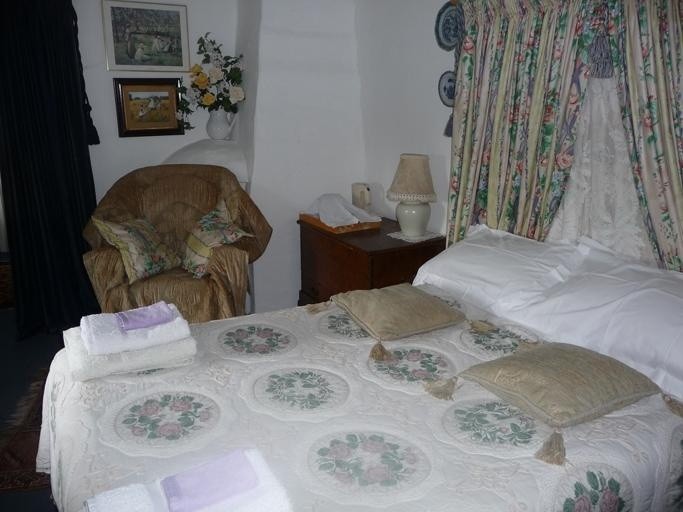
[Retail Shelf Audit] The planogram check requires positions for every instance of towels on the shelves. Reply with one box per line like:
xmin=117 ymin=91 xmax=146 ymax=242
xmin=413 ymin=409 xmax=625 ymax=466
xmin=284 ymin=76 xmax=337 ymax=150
xmin=76 ymin=445 xmax=294 ymax=511
xmin=57 ymin=300 xmax=197 ymax=384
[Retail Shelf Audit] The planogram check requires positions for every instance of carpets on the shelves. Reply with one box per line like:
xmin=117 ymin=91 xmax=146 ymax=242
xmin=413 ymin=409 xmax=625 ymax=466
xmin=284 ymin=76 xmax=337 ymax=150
xmin=0 ymin=362 xmax=57 ymax=494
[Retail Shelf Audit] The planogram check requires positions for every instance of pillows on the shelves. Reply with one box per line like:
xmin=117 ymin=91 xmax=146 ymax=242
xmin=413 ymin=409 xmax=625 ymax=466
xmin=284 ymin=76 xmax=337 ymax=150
xmin=175 ymin=195 xmax=257 ymax=282
xmin=455 ymin=338 xmax=665 ymax=430
xmin=409 ymin=223 xmax=591 ymax=318
xmin=86 ymin=211 xmax=183 ymax=288
xmin=486 ymin=232 xmax=682 ymax=406
xmin=330 ymin=281 xmax=469 ymax=345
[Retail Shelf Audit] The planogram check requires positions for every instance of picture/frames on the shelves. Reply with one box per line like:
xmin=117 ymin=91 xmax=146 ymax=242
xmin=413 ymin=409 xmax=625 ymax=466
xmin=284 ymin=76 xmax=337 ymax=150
xmin=99 ymin=0 xmax=191 ymax=73
xmin=111 ymin=77 xmax=185 ymax=139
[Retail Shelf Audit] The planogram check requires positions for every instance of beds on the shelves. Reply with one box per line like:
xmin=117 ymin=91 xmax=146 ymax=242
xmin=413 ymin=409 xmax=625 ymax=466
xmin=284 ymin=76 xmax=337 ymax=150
xmin=33 ymin=242 xmax=682 ymax=512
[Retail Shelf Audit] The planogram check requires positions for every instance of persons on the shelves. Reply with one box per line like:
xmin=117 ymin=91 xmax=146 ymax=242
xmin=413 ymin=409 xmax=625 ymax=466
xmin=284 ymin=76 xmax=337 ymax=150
xmin=133 ymin=34 xmax=180 ymax=65
xmin=136 ymin=96 xmax=163 ymax=117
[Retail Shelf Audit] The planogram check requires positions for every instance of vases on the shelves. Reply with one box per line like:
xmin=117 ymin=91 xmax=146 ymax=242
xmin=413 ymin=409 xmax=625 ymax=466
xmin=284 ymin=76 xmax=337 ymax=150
xmin=205 ymin=102 xmax=239 ymax=141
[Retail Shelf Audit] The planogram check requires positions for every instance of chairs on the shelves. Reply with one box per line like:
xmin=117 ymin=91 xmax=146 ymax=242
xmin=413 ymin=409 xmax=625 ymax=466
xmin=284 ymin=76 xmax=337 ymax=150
xmin=78 ymin=162 xmax=273 ymax=326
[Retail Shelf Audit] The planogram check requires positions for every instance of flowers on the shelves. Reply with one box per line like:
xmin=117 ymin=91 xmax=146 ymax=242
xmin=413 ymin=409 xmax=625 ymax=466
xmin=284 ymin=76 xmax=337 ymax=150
xmin=172 ymin=28 xmax=249 ymax=132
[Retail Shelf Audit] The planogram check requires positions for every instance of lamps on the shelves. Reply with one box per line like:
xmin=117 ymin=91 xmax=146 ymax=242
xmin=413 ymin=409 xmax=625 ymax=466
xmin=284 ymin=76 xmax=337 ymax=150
xmin=384 ymin=152 xmax=438 ymax=239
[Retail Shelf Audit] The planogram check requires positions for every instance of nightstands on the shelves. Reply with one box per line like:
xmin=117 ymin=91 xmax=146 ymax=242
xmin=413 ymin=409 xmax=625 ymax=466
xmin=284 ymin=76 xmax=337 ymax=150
xmin=295 ymin=211 xmax=447 ymax=310
